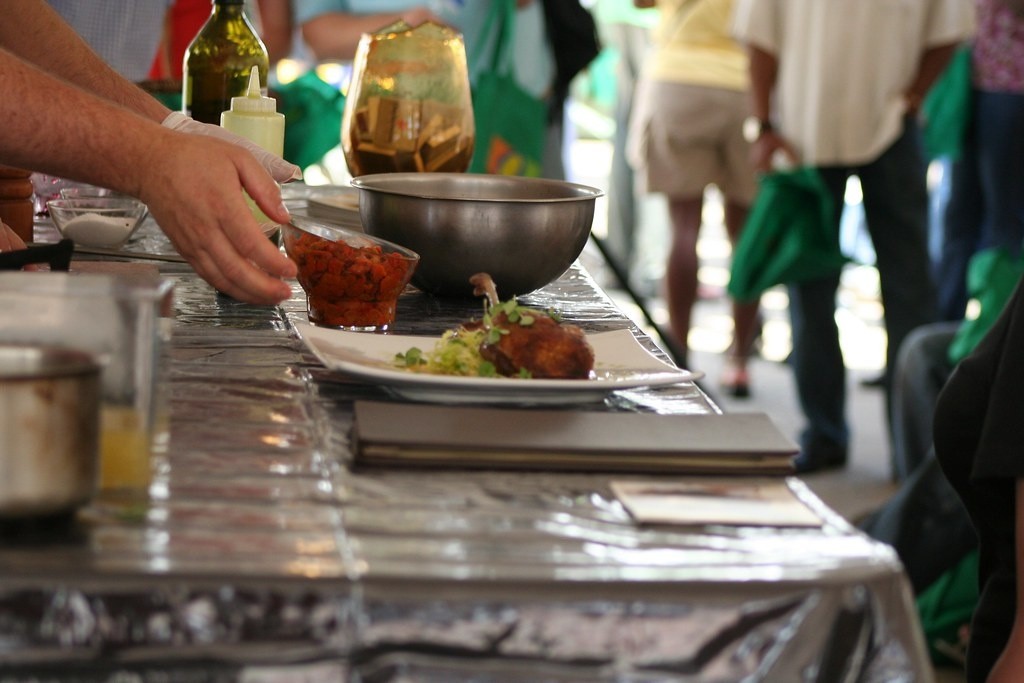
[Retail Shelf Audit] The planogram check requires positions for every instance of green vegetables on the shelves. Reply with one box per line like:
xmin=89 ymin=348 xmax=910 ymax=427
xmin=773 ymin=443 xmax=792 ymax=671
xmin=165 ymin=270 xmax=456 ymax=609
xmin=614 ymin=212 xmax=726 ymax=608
xmin=395 ymin=300 xmax=567 ymax=379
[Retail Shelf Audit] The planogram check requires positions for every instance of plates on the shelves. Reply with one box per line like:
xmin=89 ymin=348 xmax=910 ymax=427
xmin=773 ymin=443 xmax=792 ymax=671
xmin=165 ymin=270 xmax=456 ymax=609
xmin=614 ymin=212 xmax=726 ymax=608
xmin=287 ymin=322 xmax=705 ymax=405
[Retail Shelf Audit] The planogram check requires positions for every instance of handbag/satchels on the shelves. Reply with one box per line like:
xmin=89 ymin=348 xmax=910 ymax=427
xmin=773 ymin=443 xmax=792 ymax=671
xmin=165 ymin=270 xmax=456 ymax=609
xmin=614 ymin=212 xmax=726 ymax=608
xmin=467 ymin=0 xmax=547 ymax=177
xmin=727 ymin=163 xmax=864 ymax=302
xmin=917 ymin=44 xmax=974 ymax=163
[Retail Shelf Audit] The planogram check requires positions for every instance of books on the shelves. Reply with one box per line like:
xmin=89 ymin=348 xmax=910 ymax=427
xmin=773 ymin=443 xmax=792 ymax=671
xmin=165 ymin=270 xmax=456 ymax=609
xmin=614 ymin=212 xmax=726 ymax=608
xmin=353 ymin=399 xmax=802 ymax=475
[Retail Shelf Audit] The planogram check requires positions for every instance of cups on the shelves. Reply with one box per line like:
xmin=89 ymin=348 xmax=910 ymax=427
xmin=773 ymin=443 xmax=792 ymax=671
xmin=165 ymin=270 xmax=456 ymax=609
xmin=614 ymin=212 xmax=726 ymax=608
xmin=0 ymin=262 xmax=162 ymax=499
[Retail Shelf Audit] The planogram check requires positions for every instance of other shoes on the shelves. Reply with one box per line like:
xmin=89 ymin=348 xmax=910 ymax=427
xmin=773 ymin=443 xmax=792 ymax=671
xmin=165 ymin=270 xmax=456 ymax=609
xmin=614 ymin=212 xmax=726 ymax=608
xmin=790 ymin=451 xmax=849 ymax=475
xmin=717 ymin=371 xmax=751 ymax=396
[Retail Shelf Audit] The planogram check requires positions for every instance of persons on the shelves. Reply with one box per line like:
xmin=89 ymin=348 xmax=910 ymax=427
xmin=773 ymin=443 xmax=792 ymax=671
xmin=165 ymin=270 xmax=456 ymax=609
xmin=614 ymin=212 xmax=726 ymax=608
xmin=49 ymin=0 xmax=172 ymax=84
xmin=513 ymin=0 xmax=603 ymax=180
xmin=286 ymin=0 xmax=515 ymax=180
xmin=589 ymin=0 xmax=1024 ymax=683
xmin=151 ymin=0 xmax=292 ymax=87
xmin=0 ymin=0 xmax=303 ymax=304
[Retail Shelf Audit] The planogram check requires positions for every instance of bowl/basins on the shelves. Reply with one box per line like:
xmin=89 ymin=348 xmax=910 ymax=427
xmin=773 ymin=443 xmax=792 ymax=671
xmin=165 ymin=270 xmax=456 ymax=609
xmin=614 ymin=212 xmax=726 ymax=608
xmin=353 ymin=171 xmax=604 ymax=301
xmin=282 ymin=212 xmax=420 ymax=332
xmin=28 ymin=173 xmax=150 ymax=251
xmin=0 ymin=338 xmax=102 ymax=530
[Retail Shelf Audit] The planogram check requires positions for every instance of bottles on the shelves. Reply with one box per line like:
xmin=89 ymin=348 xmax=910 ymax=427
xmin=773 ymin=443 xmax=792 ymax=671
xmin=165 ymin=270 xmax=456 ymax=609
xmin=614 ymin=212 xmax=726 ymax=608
xmin=221 ymin=66 xmax=286 ymax=239
xmin=183 ymin=0 xmax=270 ymax=133
xmin=338 ymin=21 xmax=474 ymax=189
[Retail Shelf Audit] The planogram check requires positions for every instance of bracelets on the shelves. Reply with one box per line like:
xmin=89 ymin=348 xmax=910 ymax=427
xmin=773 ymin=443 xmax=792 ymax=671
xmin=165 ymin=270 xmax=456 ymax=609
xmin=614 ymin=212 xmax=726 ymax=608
xmin=758 ymin=121 xmax=773 ymax=134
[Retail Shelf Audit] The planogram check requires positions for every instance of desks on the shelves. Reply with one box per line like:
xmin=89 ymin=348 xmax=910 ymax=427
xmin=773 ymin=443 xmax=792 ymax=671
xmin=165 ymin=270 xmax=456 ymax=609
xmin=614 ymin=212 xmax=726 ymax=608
xmin=0 ymin=182 xmax=934 ymax=683
